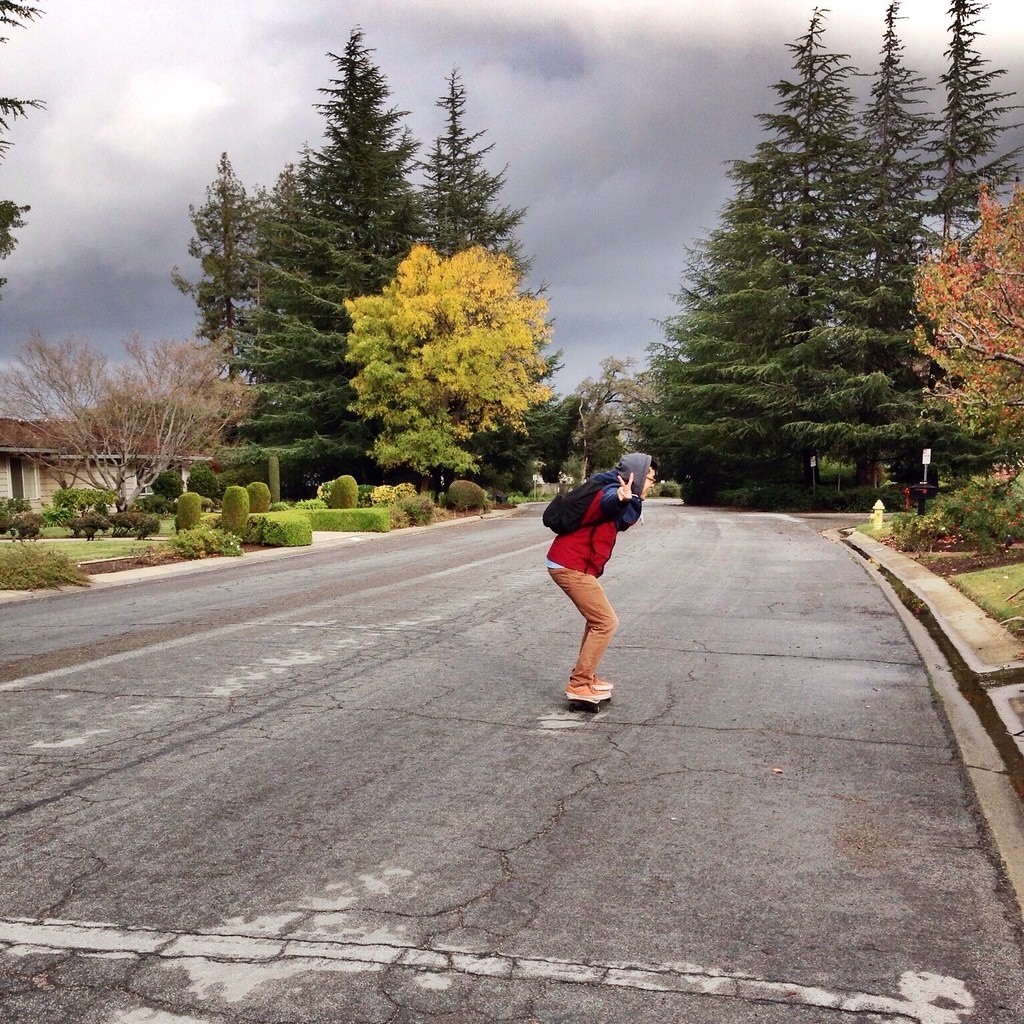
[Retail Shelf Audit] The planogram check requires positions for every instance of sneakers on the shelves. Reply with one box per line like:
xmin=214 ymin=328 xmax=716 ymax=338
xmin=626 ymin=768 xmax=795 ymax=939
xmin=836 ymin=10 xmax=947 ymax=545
xmin=591 ymin=679 xmax=614 ymax=690
xmin=565 ymin=683 xmax=612 ymax=700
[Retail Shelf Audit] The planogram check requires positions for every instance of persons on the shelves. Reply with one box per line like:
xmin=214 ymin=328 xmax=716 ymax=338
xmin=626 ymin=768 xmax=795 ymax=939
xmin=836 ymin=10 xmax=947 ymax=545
xmin=546 ymin=453 xmax=658 ymax=699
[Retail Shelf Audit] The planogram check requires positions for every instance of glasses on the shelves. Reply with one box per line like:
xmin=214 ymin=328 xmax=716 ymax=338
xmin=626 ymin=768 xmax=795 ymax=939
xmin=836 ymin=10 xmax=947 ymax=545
xmin=646 ymin=476 xmax=657 ymax=486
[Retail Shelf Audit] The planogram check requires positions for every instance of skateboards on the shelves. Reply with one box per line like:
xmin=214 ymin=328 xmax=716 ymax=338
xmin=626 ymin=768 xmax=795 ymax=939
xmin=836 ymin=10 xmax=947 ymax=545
xmin=566 ymin=690 xmax=611 ymax=713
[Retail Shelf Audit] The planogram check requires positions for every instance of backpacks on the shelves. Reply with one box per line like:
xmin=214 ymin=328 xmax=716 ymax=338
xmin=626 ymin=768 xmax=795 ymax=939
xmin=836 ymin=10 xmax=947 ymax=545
xmin=543 ymin=478 xmax=620 ymax=536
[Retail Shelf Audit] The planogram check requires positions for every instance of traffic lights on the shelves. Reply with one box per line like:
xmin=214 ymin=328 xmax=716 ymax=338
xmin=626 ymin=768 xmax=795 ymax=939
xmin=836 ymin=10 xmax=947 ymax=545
xmin=904 ymin=487 xmax=935 ymax=498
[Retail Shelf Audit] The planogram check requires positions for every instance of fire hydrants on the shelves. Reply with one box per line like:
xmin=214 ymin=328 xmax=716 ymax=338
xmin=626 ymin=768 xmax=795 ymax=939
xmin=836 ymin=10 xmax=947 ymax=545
xmin=870 ymin=500 xmax=887 ymax=532
